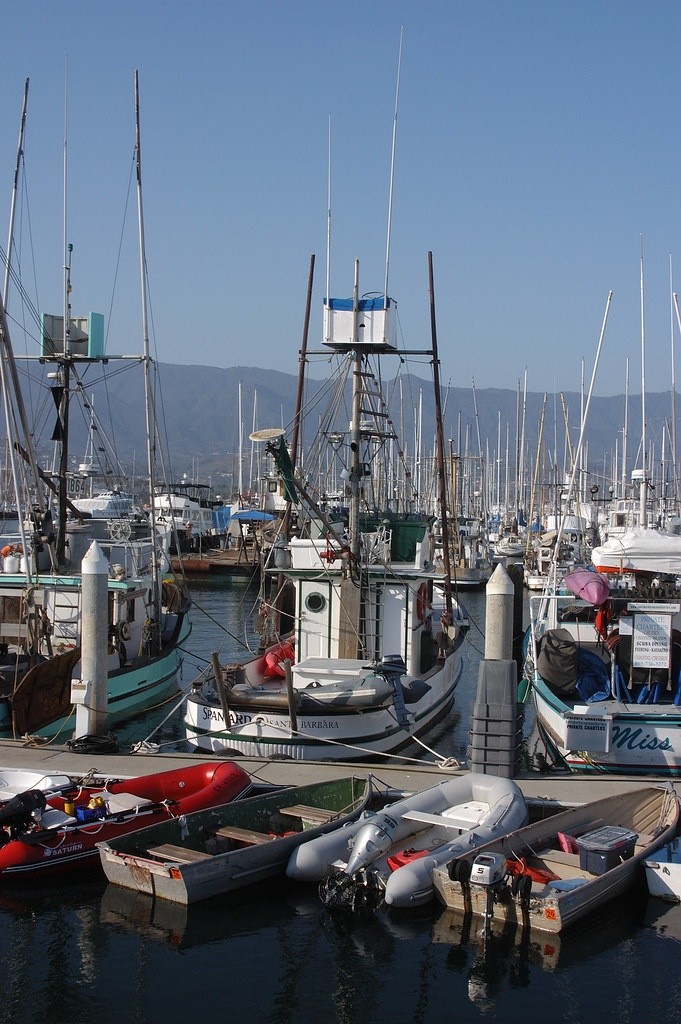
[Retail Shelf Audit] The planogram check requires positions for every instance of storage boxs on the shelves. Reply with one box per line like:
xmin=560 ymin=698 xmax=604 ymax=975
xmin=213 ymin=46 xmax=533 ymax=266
xmin=74 ymin=803 xmax=107 ymax=822
xmin=575 ymin=826 xmax=638 ymax=875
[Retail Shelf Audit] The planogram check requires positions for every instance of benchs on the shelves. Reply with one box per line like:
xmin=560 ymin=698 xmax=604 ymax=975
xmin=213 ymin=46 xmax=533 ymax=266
xmin=279 ymin=804 xmax=350 ymax=823
xmin=31 ymin=805 xmax=78 ymax=830
xmin=135 ymin=843 xmax=212 ymax=863
xmin=204 ymin=825 xmax=277 ymax=846
xmin=89 ymin=792 xmax=115 ymax=802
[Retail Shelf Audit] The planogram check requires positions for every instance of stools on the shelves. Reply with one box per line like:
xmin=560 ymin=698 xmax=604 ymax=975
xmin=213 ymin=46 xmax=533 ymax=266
xmin=548 ymin=879 xmax=589 ymax=894
xmin=441 ymin=800 xmax=490 ymax=822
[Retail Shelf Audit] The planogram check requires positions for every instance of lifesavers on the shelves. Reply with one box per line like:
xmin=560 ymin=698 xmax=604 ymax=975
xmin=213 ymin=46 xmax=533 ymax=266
xmin=1 ymin=543 xmax=23 ymax=559
xmin=417 ymin=582 xmax=426 ymax=620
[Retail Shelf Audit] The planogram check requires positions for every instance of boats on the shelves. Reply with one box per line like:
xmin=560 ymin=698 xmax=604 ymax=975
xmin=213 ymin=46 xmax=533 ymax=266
xmin=432 ymin=778 xmax=681 ymax=936
xmin=99 ymin=880 xmax=310 ymax=949
xmin=641 ymin=834 xmax=681 ymax=904
xmin=0 ymin=767 xmax=84 ymax=813
xmin=94 ymin=772 xmax=373 ymax=910
xmin=0 ymin=760 xmax=256 ymax=880
xmin=433 ymin=880 xmax=652 ymax=975
xmin=286 ymin=768 xmax=530 ymax=915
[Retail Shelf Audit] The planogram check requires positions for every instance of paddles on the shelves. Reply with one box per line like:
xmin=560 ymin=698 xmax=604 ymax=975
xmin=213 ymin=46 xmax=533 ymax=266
xmin=17 ymin=798 xmax=177 ymax=846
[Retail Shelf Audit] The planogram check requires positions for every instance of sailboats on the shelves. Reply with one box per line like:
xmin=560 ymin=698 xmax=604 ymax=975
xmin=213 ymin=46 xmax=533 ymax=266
xmin=1 ymin=29 xmax=681 ymax=781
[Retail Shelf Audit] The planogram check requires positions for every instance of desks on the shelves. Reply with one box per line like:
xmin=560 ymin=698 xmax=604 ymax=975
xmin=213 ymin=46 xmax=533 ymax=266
xmin=404 ymin=810 xmax=480 ymax=835
